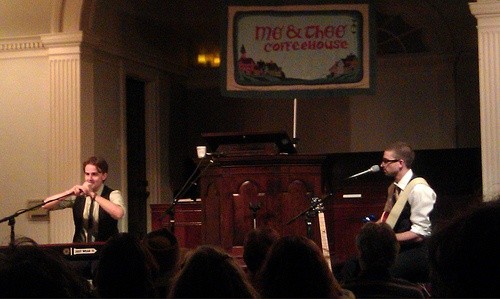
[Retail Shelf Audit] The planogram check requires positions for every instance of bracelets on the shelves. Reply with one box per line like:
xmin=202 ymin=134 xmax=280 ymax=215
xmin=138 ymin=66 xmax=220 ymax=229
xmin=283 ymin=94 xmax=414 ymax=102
xmin=93 ymin=193 xmax=97 ymax=201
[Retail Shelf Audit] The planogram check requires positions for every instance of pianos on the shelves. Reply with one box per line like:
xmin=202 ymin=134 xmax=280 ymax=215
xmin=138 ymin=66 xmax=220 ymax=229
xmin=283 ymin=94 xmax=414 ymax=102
xmin=1 ymin=241 xmax=108 ymax=262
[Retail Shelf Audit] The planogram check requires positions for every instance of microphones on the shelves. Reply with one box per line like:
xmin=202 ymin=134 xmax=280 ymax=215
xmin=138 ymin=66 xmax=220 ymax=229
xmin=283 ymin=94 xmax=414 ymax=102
xmin=346 ymin=165 xmax=380 ymax=180
xmin=206 ymin=152 xmax=225 ymax=157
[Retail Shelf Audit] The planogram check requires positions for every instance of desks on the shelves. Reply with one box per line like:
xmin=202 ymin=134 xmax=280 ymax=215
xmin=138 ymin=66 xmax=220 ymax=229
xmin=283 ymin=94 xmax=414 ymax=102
xmin=149 ymin=129 xmax=385 ymax=272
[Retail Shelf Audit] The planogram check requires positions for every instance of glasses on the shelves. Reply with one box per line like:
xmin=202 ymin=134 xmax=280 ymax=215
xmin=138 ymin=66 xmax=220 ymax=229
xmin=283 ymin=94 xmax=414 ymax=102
xmin=383 ymin=159 xmax=401 ymax=164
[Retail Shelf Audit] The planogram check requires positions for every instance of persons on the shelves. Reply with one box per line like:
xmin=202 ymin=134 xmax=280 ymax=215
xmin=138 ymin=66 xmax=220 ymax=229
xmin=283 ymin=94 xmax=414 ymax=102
xmin=370 ymin=142 xmax=437 ymax=284
xmin=41 ymin=156 xmax=126 ymax=243
xmin=0 ymin=198 xmax=500 ymax=299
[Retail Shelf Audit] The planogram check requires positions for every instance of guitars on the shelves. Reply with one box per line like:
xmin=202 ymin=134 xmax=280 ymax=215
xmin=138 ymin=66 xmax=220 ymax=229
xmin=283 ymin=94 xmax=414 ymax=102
xmin=312 ymin=196 xmax=332 ymax=274
xmin=358 ymin=213 xmax=378 ymax=224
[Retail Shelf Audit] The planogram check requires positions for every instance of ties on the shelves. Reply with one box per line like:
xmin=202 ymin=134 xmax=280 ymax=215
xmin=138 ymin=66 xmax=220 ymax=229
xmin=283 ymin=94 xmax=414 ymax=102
xmin=397 ymin=187 xmax=401 ymax=195
xmin=87 ymin=198 xmax=95 ymax=232
